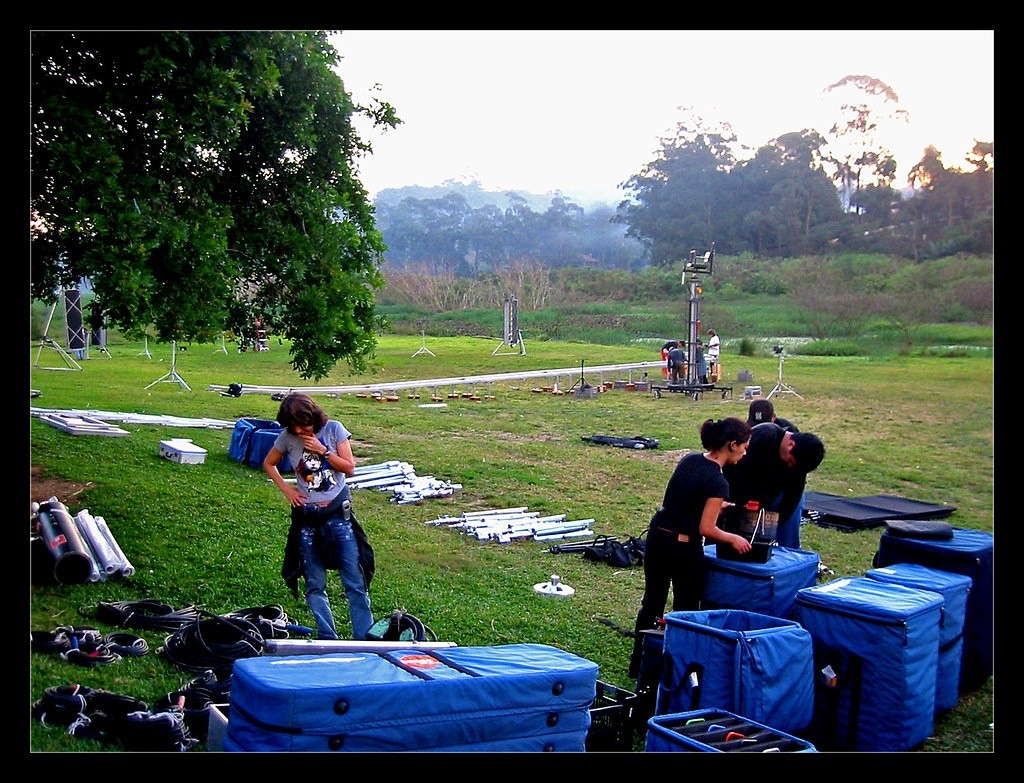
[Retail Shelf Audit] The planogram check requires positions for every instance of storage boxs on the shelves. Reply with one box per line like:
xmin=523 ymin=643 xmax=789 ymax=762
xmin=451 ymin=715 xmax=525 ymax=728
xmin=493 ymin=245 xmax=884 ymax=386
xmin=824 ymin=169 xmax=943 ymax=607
xmin=208 ymin=703 xmax=229 ymax=752
xmin=635 ymin=382 xmax=649 ymax=391
xmin=738 ymin=370 xmax=754 ymax=382
xmin=582 ymin=678 xmax=640 ymax=752
xmin=714 ymin=538 xmax=776 ymax=564
xmin=159 ymin=439 xmax=208 ymax=464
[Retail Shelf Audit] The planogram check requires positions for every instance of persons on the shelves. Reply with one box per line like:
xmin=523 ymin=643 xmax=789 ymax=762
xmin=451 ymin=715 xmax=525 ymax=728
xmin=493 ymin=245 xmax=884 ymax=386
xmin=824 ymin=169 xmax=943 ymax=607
xmin=629 ymin=415 xmax=752 ymax=678
xmin=661 ymin=329 xmax=721 ymax=382
xmin=723 ymin=398 xmax=828 ymax=552
xmin=263 ymin=392 xmax=377 ymax=640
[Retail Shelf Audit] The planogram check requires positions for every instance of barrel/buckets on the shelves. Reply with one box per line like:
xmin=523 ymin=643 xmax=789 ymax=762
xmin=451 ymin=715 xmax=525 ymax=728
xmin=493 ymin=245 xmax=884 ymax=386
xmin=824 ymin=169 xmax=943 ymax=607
xmin=727 ymin=506 xmax=780 ymax=541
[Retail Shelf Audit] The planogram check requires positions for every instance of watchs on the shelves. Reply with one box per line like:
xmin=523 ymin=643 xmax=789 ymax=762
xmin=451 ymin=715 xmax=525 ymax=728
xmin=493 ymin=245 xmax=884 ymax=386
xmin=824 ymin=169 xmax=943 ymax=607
xmin=321 ymin=444 xmax=331 ymax=459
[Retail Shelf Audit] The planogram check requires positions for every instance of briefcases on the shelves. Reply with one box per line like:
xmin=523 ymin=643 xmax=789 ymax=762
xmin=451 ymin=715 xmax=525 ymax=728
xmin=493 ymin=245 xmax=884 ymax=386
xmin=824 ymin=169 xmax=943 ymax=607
xmin=159 ymin=439 xmax=208 ymax=465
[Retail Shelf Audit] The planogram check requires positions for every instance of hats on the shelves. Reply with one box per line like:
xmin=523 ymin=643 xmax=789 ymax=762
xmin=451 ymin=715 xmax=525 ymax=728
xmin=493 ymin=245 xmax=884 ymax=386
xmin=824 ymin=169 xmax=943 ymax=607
xmin=747 ymin=400 xmax=773 ymax=424
